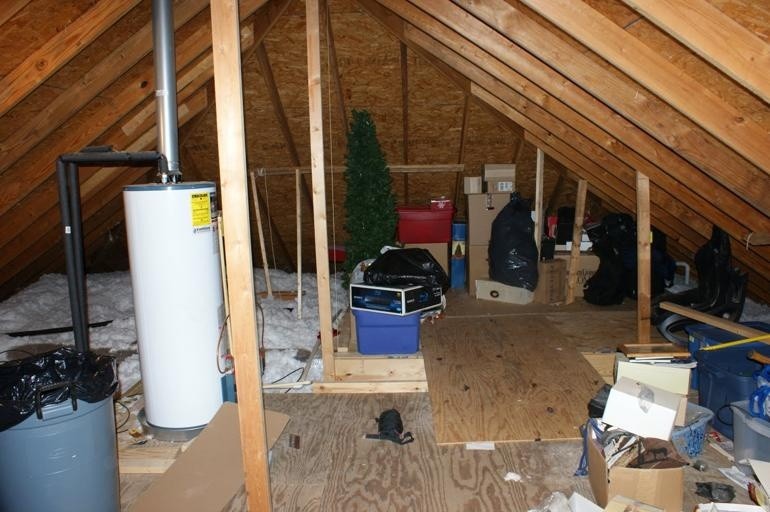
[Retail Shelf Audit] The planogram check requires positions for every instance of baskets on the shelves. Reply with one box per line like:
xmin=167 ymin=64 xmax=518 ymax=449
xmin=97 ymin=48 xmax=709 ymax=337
xmin=671 ymin=402 xmax=715 ymax=459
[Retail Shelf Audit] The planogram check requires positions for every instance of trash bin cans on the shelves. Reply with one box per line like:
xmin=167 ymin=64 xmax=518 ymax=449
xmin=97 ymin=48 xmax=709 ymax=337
xmin=0 ymin=349 xmax=120 ymax=512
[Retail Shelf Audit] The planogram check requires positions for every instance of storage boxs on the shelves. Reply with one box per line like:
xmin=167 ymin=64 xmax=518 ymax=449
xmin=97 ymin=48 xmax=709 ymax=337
xmin=348 ymin=284 xmax=442 ymax=355
xmin=728 ymin=399 xmax=770 ymax=480
xmin=396 ymin=165 xmax=602 ymax=307
xmin=584 ymin=416 xmax=691 ymax=512
